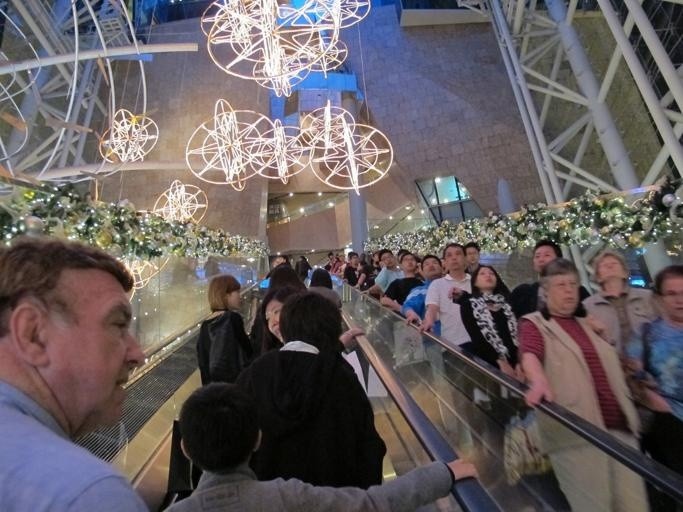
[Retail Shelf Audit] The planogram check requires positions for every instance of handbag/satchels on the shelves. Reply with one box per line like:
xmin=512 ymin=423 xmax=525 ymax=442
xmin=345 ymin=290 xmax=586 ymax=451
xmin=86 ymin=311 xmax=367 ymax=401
xmin=502 ymin=412 xmax=551 ymax=487
xmin=206 ymin=311 xmax=247 ymax=379
xmin=168 ymin=421 xmax=202 ymax=493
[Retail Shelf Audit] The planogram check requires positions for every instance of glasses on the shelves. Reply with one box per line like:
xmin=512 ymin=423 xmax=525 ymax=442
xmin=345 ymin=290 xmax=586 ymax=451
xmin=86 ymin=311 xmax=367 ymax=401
xmin=660 ymin=292 xmax=683 ymax=301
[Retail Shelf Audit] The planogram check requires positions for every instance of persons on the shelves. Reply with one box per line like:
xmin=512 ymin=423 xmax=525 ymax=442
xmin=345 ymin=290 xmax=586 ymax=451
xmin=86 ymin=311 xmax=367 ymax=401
xmin=623 ymin=263 xmax=683 ymax=511
xmin=225 ymin=290 xmax=385 ymax=490
xmin=0 ymin=236 xmax=157 ymax=512
xmin=324 ymin=240 xmax=655 ymax=452
xmin=194 ymin=254 xmax=365 ymax=387
xmin=157 ymin=377 xmax=481 ymax=512
xmin=514 ymin=253 xmax=651 ymax=512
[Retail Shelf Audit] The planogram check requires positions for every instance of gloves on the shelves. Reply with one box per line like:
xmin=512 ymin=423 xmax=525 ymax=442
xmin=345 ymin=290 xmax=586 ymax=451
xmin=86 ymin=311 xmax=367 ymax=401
xmin=360 ymin=290 xmax=369 ymax=296
xmin=354 ymin=284 xmax=360 ymax=289
